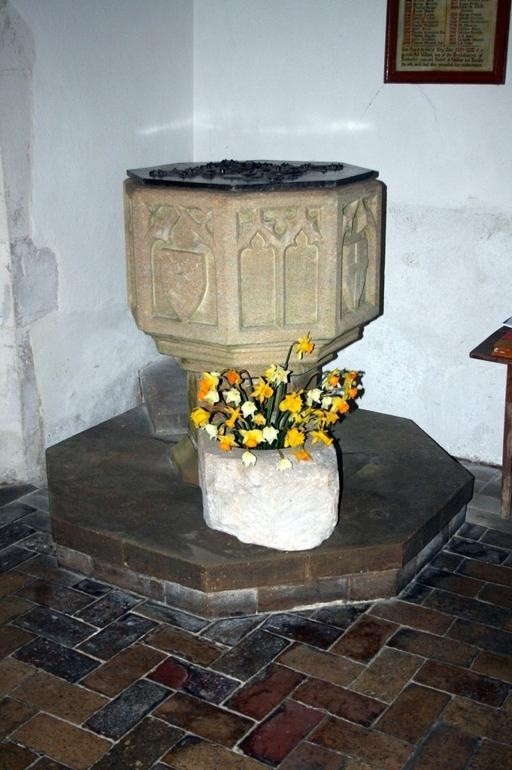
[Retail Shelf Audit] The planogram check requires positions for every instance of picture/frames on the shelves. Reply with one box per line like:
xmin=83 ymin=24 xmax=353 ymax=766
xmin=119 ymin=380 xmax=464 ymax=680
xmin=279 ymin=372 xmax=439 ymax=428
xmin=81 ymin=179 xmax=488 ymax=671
xmin=382 ymin=0 xmax=512 ymax=85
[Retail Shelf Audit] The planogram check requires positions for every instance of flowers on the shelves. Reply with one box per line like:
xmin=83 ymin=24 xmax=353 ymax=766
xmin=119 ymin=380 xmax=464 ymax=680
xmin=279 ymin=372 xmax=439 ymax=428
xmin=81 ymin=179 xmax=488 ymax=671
xmin=190 ymin=330 xmax=366 ymax=473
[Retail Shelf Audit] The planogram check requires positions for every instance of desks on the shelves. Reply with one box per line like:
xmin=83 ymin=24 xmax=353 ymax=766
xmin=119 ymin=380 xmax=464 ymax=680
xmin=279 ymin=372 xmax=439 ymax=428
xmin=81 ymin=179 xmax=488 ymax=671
xmin=468 ymin=326 xmax=512 ymax=522
